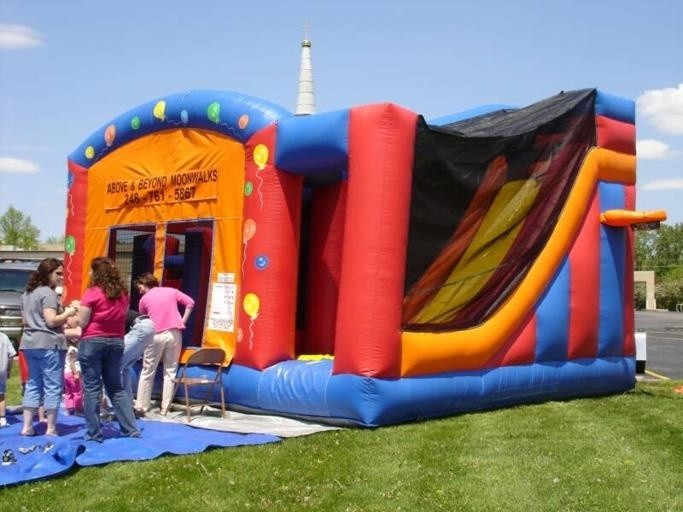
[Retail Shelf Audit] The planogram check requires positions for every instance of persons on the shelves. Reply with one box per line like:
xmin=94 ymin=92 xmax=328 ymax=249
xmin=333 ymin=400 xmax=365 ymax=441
xmin=16 ymin=256 xmax=156 ymax=442
xmin=133 ymin=271 xmax=194 ymax=416
xmin=0 ymin=331 xmax=17 ymax=428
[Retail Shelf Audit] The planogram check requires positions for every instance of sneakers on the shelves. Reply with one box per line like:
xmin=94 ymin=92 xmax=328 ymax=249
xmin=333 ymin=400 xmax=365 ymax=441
xmin=2 ymin=450 xmax=17 ymax=465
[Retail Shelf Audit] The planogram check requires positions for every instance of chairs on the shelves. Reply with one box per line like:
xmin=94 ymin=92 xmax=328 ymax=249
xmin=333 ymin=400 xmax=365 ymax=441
xmin=160 ymin=349 xmax=226 ymax=423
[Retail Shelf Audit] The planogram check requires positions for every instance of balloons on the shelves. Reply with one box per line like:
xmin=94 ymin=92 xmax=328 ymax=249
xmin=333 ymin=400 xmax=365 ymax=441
xmin=207 ymin=104 xmax=241 ymax=138
xmin=253 ymin=144 xmax=269 ymax=209
xmin=67 ymin=171 xmax=75 ymax=216
xmin=241 ymin=218 xmax=256 ymax=278
xmin=64 ymin=235 xmax=76 ymax=286
xmin=153 ymin=99 xmax=183 ymax=127
xmin=95 ymin=123 xmax=116 ymax=162
xmin=243 ymin=293 xmax=259 ymax=350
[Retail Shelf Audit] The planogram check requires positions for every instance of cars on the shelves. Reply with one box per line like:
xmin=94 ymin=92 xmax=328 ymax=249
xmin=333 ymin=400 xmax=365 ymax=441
xmin=0 ymin=261 xmax=39 ymax=338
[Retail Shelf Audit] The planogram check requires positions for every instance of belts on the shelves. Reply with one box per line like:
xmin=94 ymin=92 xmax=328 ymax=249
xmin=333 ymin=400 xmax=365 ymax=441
xmin=130 ymin=316 xmax=149 ymax=327
